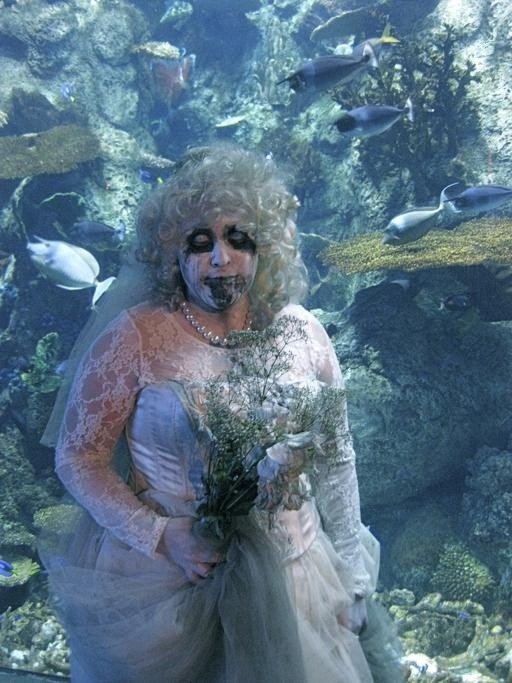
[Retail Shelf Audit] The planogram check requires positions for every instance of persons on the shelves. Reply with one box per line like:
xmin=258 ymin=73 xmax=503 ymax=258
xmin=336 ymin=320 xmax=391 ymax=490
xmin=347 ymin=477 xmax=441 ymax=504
xmin=32 ymin=140 xmax=407 ymax=683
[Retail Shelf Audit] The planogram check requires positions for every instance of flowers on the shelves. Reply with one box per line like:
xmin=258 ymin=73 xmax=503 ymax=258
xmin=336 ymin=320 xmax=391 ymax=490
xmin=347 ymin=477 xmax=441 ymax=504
xmin=167 ymin=317 xmax=353 ymax=595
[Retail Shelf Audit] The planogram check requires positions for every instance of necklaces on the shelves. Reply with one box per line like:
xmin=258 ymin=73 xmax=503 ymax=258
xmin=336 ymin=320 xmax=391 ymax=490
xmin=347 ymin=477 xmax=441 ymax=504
xmin=179 ymin=299 xmax=255 ymax=346
